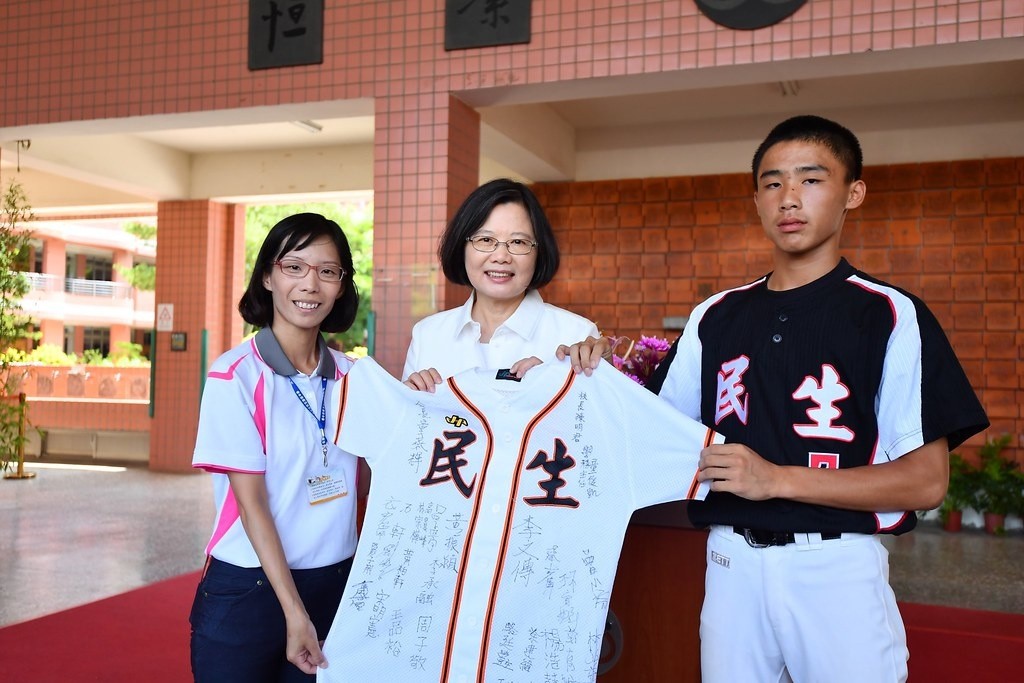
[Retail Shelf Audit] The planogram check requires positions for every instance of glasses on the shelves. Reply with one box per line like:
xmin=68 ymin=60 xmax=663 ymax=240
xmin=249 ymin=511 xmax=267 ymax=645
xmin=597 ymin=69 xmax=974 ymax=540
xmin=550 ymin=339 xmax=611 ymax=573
xmin=466 ymin=234 xmax=538 ymax=255
xmin=272 ymin=258 xmax=347 ymax=283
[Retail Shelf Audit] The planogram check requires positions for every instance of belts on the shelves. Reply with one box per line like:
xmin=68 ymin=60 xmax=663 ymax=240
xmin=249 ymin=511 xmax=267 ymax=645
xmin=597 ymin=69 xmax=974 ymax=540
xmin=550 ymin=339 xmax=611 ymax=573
xmin=712 ymin=523 xmax=867 ymax=549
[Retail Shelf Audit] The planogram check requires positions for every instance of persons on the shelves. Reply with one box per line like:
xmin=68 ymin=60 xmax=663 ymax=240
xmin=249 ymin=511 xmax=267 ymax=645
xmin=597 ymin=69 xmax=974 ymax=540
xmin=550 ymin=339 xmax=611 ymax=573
xmin=400 ymin=178 xmax=600 ymax=683
xmin=189 ymin=213 xmax=364 ymax=683
xmin=556 ymin=114 xmax=990 ymax=683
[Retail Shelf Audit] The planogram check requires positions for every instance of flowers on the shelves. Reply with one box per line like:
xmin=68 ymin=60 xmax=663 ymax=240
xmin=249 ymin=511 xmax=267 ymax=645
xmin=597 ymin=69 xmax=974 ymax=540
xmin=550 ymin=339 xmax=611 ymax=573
xmin=610 ymin=332 xmax=679 ymax=391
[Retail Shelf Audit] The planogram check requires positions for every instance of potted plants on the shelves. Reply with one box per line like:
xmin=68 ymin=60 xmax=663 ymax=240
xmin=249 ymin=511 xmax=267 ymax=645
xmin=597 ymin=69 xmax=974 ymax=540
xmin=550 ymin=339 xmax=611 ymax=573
xmin=917 ymin=453 xmax=980 ymax=532
xmin=970 ymin=433 xmax=1024 ymax=534
